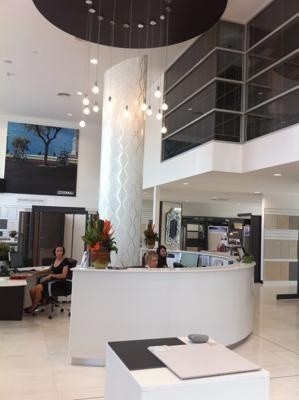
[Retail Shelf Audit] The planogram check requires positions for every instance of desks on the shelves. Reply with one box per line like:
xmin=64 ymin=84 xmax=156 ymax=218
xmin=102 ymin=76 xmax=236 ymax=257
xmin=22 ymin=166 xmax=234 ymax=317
xmin=0 ymin=266 xmax=52 ymax=321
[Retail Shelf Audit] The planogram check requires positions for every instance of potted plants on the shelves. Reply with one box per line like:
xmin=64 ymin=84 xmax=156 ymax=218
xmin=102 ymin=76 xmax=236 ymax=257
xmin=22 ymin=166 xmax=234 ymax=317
xmin=9 ymin=230 xmax=17 ymax=242
xmin=144 ymin=224 xmax=159 ymax=249
xmin=81 ymin=219 xmax=118 ymax=269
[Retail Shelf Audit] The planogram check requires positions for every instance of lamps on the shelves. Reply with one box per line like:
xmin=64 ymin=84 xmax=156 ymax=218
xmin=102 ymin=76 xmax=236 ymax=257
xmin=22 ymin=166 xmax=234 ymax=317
xmin=76 ymin=0 xmax=170 ymax=134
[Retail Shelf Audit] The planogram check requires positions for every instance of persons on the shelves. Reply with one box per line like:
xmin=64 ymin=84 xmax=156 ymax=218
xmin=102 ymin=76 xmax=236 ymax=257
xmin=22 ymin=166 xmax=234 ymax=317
xmin=144 ymin=250 xmax=158 ymax=268
xmin=24 ymin=245 xmax=71 ymax=313
xmin=157 ymin=245 xmax=169 ymax=268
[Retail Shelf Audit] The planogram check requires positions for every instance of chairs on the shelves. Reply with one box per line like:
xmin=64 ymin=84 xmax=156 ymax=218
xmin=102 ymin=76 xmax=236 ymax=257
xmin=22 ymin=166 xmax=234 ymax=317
xmin=32 ymin=258 xmax=78 ymax=319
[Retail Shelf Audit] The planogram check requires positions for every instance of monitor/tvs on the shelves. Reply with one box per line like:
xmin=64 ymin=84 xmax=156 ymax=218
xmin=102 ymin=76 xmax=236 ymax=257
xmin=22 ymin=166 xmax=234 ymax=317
xmin=9 ymin=252 xmax=24 ymax=272
xmin=180 ymin=254 xmax=199 ymax=267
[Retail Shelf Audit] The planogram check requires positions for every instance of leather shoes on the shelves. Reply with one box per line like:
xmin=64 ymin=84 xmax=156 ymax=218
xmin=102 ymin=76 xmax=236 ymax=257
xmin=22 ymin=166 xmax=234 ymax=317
xmin=24 ymin=303 xmax=40 ymax=313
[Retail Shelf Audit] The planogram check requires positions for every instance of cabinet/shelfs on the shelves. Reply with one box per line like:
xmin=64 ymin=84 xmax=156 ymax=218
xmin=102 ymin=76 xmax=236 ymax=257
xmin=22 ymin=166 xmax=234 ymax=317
xmin=180 ymin=215 xmax=262 ymax=283
